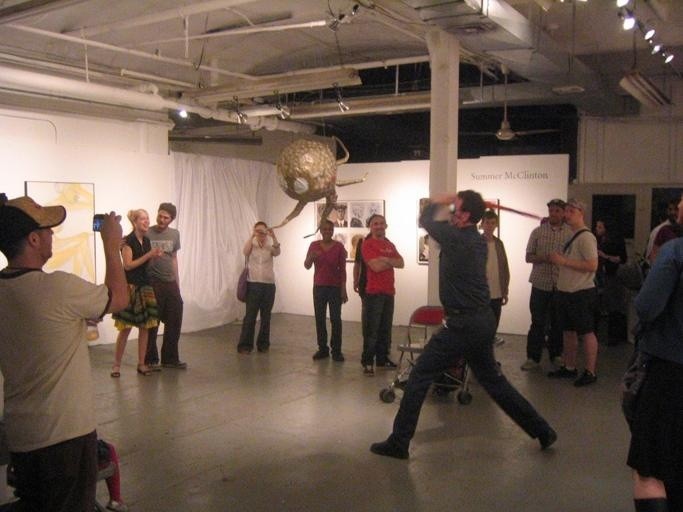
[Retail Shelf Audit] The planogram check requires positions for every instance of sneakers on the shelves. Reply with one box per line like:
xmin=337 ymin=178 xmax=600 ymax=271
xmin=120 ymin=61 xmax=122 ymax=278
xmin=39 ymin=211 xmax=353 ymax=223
xmin=371 ymin=436 xmax=409 ymax=459
xmin=574 ymin=370 xmax=596 ymax=386
xmin=146 ymin=360 xmax=161 ymax=371
xmin=549 ymin=366 xmax=577 ymax=378
xmin=106 ymin=499 xmax=128 ymax=511
xmin=257 ymin=344 xmax=269 ymax=353
xmin=376 ymin=358 xmax=397 ymax=369
xmin=521 ymin=359 xmax=543 ymax=370
xmin=238 ymin=345 xmax=251 ymax=354
xmin=312 ymin=350 xmax=329 ymax=359
xmin=332 ymin=354 xmax=344 ymax=360
xmin=540 ymin=428 xmax=557 ymax=450
xmin=161 ymin=359 xmax=188 ymax=368
xmin=363 ymin=365 xmax=374 ymax=376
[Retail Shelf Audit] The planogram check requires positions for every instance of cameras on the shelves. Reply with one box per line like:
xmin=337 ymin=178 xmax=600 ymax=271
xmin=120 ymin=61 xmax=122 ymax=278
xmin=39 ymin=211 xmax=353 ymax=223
xmin=93 ymin=215 xmax=109 ymax=233
xmin=257 ymin=229 xmax=268 ymax=235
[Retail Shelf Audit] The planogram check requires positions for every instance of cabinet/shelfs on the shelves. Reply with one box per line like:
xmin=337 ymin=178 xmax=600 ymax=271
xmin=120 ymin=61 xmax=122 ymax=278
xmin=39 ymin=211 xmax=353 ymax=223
xmin=577 ymin=116 xmax=683 ymax=184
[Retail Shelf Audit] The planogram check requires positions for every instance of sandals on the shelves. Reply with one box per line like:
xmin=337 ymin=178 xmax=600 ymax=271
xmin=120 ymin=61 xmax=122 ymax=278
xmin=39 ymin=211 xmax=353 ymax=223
xmin=137 ymin=364 xmax=152 ymax=375
xmin=111 ymin=365 xmax=120 ymax=377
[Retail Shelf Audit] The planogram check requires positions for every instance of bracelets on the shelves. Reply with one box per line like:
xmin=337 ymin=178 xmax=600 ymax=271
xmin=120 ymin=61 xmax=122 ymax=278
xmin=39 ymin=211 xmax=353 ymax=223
xmin=272 ymin=242 xmax=280 ymax=248
xmin=564 ymin=256 xmax=570 ymax=266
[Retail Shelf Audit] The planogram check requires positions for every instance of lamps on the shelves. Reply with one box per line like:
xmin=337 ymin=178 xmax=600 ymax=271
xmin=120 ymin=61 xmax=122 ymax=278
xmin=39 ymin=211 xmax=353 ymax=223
xmin=613 ymin=66 xmax=670 ymax=110
xmin=189 ymin=64 xmax=366 ymax=98
xmin=1 ymin=55 xmax=166 ymax=113
xmin=610 ymin=0 xmax=675 ymax=62
xmin=227 ymin=101 xmax=361 ymax=127
xmin=496 ymin=69 xmax=517 ymax=141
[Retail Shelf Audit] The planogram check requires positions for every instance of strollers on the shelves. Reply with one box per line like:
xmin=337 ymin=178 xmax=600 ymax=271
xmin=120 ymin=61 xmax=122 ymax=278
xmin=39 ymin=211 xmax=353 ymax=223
xmin=379 ymin=305 xmax=472 ymax=405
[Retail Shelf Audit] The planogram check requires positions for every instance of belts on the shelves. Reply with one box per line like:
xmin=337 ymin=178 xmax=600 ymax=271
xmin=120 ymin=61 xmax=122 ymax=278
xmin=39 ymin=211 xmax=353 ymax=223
xmin=446 ymin=306 xmax=492 ymax=314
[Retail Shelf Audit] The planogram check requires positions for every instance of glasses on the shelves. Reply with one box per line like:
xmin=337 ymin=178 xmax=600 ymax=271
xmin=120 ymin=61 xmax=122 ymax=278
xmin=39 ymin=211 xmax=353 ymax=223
xmin=448 ymin=203 xmax=456 ymax=215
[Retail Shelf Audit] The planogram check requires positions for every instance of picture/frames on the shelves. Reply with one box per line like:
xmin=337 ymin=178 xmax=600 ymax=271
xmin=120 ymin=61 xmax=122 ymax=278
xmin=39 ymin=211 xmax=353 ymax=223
xmin=313 ymin=198 xmax=384 ymax=260
xmin=24 ymin=181 xmax=98 ymax=285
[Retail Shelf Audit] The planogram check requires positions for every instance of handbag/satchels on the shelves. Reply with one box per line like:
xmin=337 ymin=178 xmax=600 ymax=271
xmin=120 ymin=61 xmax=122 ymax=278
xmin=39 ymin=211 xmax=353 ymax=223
xmin=595 ymin=257 xmax=613 ymax=286
xmin=237 ymin=268 xmax=249 ymax=303
xmin=616 ymin=258 xmax=645 ymax=290
xmin=621 ymin=353 xmax=652 ymax=430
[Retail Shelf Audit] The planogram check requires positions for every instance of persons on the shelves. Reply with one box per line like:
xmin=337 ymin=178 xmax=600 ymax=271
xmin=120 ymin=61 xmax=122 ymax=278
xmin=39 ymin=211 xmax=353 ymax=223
xmin=481 ymin=210 xmax=511 ymax=337
xmin=96 ymin=438 xmax=130 ymax=511
xmin=317 ymin=202 xmax=382 ymax=260
xmin=236 ymin=221 xmax=281 ymax=355
xmin=110 ymin=207 xmax=165 ymax=377
xmin=304 ymin=219 xmax=349 ymax=361
xmin=520 ymin=197 xmax=574 ymax=370
xmin=548 ymin=198 xmax=600 ymax=386
xmin=144 ymin=202 xmax=188 ymax=371
xmin=0 ymin=194 xmax=131 ymax=512
xmin=370 ymin=188 xmax=558 ymax=460
xmin=591 ymin=213 xmax=627 ymax=347
xmin=614 ymin=193 xmax=683 ymax=511
xmin=360 ymin=214 xmax=405 ymax=377
xmin=352 ymin=213 xmax=394 ymax=366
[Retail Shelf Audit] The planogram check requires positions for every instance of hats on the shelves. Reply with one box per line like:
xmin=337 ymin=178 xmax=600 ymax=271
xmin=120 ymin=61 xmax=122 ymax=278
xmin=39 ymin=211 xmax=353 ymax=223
xmin=547 ymin=199 xmax=565 ymax=209
xmin=560 ymin=198 xmax=586 ymax=211
xmin=1 ymin=196 xmax=66 ymax=250
